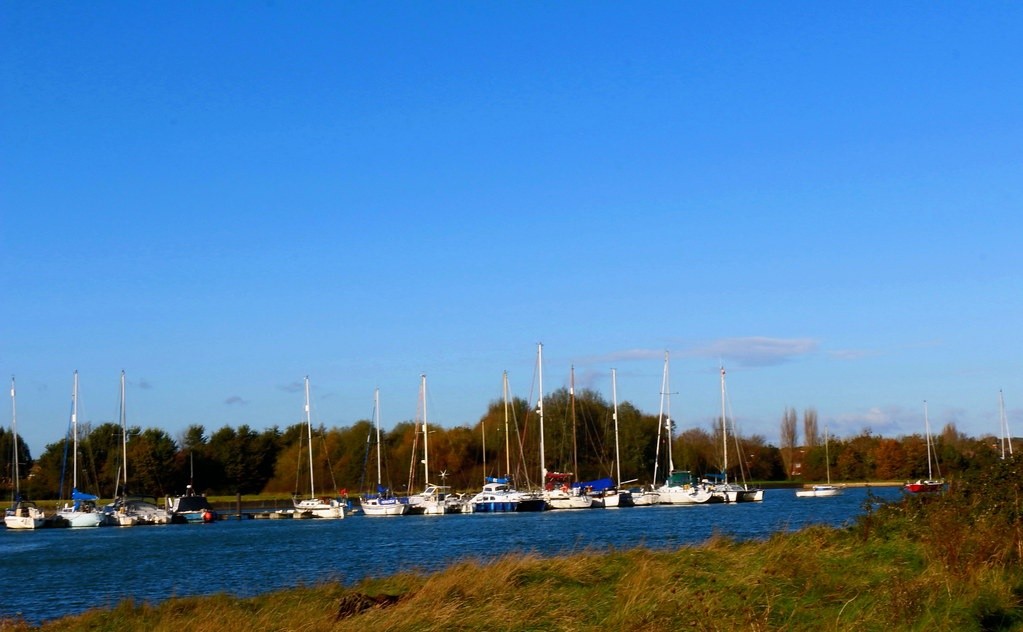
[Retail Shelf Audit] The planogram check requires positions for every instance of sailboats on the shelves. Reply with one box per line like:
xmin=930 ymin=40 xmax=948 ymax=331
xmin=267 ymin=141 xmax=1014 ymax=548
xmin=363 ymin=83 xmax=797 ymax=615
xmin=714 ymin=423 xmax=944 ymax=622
xmin=795 ymin=424 xmax=842 ymax=498
xmin=904 ymin=399 xmax=946 ymax=494
xmin=50 ymin=369 xmax=218 ymax=527
xmin=4 ymin=372 xmax=47 ymax=529
xmin=359 ymin=340 xmax=764 ymax=516
xmin=291 ymin=374 xmax=348 ymax=520
